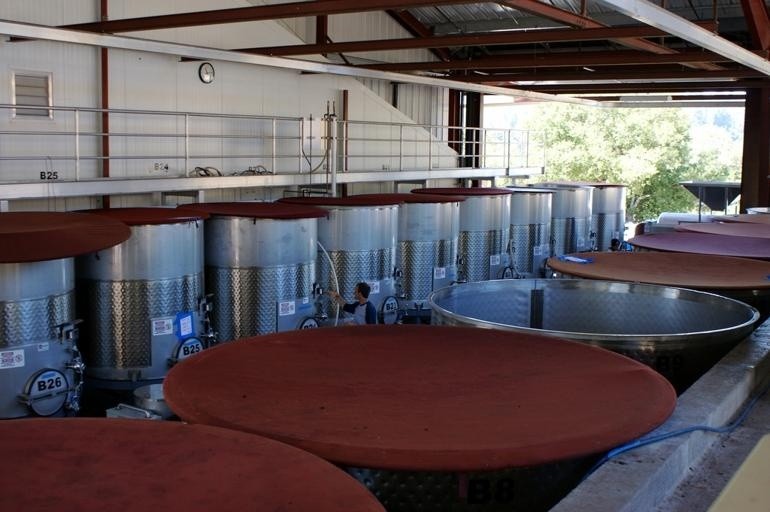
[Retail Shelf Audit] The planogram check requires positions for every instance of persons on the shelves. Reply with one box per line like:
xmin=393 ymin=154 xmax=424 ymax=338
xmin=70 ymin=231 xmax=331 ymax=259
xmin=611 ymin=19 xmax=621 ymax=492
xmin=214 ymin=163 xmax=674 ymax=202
xmin=329 ymin=283 xmax=376 ymax=324
xmin=608 ymin=239 xmax=620 ymax=251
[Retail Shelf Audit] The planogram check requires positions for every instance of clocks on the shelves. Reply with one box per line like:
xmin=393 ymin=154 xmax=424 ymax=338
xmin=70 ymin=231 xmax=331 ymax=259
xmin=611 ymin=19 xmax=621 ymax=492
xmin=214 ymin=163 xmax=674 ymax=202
xmin=198 ymin=61 xmax=215 ymax=83
